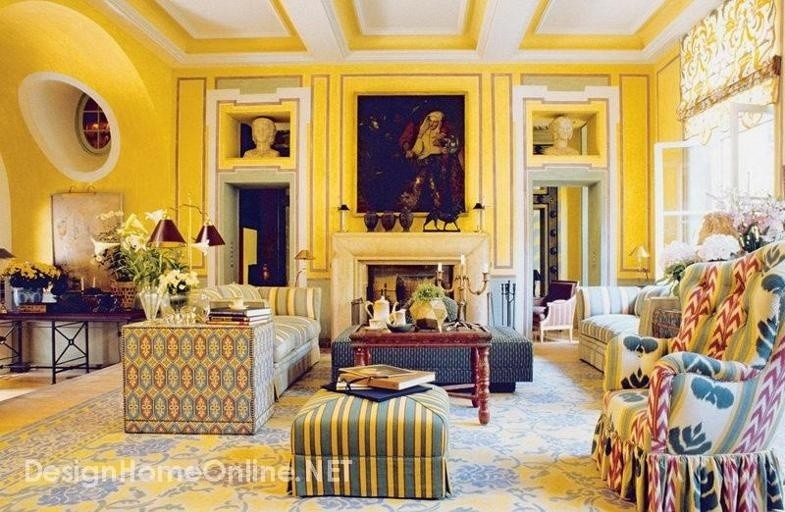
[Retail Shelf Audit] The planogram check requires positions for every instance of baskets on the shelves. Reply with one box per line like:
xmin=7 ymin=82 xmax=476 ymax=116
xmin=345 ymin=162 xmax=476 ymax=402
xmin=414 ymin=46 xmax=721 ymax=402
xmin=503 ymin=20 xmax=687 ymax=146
xmin=109 ymin=281 xmax=136 ymax=311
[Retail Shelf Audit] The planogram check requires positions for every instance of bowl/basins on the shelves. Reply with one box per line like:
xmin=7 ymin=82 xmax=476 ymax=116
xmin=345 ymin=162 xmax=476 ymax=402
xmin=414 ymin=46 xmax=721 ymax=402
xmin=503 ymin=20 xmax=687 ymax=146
xmin=386 ymin=323 xmax=412 ymax=332
xmin=80 ymin=294 xmax=124 ymax=313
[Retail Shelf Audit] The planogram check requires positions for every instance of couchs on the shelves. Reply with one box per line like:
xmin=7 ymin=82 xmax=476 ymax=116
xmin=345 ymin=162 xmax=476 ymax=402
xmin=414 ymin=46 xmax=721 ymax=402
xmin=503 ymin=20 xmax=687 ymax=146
xmin=331 ymin=322 xmax=537 ymax=393
xmin=286 ymin=385 xmax=453 ymax=504
xmin=570 ymin=277 xmax=679 ymax=376
xmin=118 ymin=281 xmax=324 ymax=440
xmin=584 ymin=242 xmax=785 ymax=511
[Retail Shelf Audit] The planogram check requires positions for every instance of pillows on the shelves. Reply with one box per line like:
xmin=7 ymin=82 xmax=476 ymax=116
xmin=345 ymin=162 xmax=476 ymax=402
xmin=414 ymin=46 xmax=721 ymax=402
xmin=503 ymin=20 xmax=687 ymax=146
xmin=632 ymin=283 xmax=673 ymax=318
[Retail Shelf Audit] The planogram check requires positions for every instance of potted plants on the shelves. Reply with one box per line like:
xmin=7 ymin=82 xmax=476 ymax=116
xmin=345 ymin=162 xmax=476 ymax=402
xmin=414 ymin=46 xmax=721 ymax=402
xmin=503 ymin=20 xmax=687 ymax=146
xmin=409 ymin=279 xmax=454 ymax=333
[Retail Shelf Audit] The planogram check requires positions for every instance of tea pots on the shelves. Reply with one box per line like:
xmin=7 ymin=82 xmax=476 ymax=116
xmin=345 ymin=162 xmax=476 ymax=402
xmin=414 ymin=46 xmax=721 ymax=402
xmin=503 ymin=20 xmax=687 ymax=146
xmin=365 ymin=296 xmax=399 ymax=319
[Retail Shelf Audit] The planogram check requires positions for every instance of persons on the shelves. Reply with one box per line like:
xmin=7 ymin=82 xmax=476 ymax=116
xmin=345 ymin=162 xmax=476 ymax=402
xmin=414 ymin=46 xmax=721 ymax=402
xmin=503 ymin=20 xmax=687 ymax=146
xmin=242 ymin=117 xmax=281 ymax=158
xmin=542 ymin=116 xmax=579 ymax=156
xmin=397 ymin=111 xmax=450 ymax=210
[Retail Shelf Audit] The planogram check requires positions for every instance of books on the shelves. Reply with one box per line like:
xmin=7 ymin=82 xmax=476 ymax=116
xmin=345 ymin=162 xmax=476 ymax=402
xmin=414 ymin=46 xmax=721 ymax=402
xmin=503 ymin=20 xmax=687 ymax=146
xmin=336 ymin=377 xmax=373 ymax=391
xmin=205 ymin=298 xmax=271 ymax=326
xmin=339 ymin=370 xmax=437 ymax=391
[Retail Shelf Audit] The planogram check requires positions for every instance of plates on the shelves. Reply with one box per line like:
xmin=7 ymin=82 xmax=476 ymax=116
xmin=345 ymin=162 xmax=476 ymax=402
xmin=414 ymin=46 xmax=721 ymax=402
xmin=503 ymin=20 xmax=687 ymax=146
xmin=365 ymin=327 xmax=383 ymax=331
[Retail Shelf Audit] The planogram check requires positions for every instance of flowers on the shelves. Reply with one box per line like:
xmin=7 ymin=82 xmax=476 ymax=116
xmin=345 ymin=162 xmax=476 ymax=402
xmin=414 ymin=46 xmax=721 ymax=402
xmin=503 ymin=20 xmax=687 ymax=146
xmin=0 ymin=259 xmax=65 ymax=282
xmin=88 ymin=209 xmax=137 ymax=280
xmin=117 ymin=205 xmax=204 ymax=293
xmin=664 ymin=188 xmax=783 ymax=280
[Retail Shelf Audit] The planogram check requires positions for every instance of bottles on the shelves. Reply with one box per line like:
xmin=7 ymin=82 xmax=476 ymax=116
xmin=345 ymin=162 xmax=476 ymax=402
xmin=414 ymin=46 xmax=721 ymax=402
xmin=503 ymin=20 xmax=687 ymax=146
xmin=363 ymin=210 xmax=378 ymax=232
xmin=381 ymin=210 xmax=396 ymax=231
xmin=398 ymin=210 xmax=413 ymax=232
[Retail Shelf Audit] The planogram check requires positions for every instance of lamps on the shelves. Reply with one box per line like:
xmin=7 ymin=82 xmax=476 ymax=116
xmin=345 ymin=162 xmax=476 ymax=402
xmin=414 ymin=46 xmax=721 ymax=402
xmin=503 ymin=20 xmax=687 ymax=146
xmin=293 ymin=247 xmax=314 ymax=269
xmin=146 ymin=197 xmax=228 ymax=270
xmin=632 ymin=242 xmax=647 ymax=273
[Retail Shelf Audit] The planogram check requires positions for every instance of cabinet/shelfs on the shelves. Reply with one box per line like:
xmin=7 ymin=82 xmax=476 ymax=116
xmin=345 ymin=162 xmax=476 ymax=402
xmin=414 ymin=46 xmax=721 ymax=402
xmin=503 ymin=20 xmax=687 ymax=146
xmin=652 ymin=308 xmax=684 ymax=338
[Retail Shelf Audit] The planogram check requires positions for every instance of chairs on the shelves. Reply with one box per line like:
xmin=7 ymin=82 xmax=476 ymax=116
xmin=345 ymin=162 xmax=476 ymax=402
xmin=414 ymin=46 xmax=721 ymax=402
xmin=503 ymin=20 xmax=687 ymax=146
xmin=533 ymin=275 xmax=580 ymax=345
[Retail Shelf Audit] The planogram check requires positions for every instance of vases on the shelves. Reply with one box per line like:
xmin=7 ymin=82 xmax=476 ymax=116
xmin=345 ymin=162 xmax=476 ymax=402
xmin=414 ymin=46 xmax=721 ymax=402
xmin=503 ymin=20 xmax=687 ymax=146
xmin=109 ymin=278 xmax=137 ymax=313
xmin=363 ymin=208 xmax=414 ymax=233
xmin=139 ymin=285 xmax=162 ymax=322
xmin=12 ymin=284 xmax=49 ymax=306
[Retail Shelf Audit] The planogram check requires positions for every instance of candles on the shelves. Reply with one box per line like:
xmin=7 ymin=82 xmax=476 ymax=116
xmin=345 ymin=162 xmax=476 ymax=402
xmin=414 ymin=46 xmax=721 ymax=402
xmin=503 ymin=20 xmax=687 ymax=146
xmin=438 ymin=254 xmax=496 ymax=333
xmin=78 ymin=274 xmax=89 ymax=293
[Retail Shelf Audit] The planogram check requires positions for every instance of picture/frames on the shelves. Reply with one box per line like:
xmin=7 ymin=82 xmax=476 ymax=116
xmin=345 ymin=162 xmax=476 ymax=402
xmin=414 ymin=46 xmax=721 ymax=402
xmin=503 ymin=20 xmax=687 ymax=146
xmin=352 ymin=87 xmax=471 ymax=218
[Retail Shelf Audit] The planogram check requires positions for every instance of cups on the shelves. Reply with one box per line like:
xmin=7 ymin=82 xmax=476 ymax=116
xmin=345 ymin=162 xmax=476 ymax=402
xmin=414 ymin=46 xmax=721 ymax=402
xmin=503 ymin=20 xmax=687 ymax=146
xmin=232 ymin=297 xmax=243 ymax=307
xmin=369 ymin=320 xmax=380 ymax=328
xmin=393 ymin=311 xmax=406 ymax=325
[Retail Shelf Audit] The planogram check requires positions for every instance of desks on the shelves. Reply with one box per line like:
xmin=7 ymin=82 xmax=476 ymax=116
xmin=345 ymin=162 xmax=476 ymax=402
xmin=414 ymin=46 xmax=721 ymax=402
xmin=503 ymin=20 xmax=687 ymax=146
xmin=347 ymin=324 xmax=501 ymax=426
xmin=0 ymin=307 xmax=124 ymax=387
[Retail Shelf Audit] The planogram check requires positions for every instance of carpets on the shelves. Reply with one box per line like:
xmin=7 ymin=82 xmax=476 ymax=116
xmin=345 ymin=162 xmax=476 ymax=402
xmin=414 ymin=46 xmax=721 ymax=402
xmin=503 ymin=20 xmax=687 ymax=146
xmin=0 ymin=360 xmax=642 ymax=511
